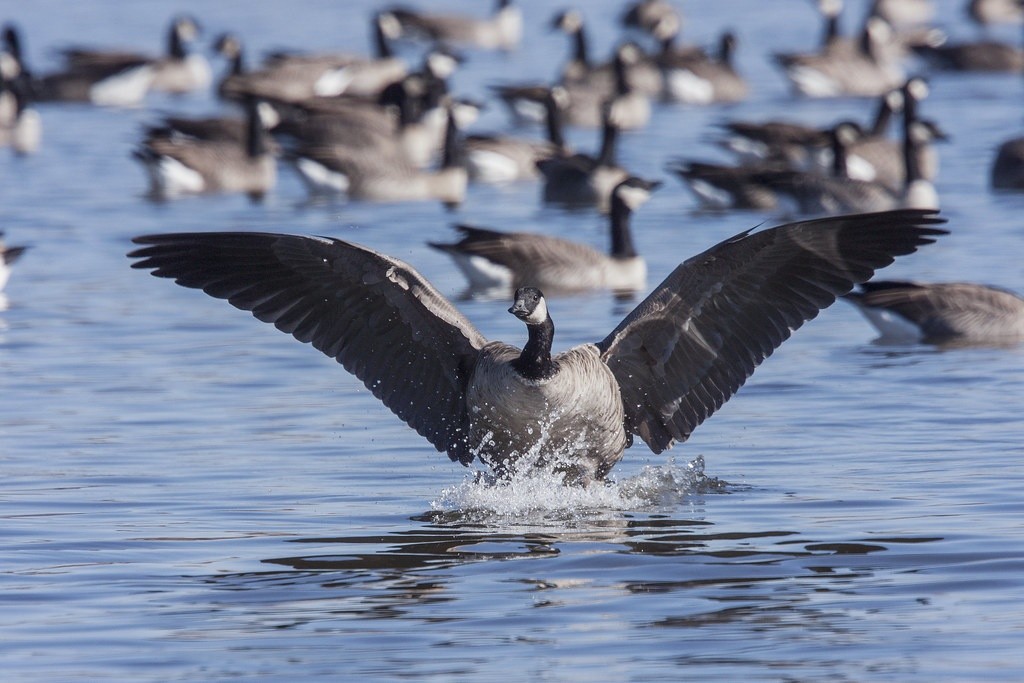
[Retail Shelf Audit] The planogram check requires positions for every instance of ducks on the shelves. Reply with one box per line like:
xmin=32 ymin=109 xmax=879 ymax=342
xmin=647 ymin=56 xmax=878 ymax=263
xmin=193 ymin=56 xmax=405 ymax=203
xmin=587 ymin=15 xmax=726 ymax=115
xmin=2 ymin=4 xmax=1022 ymax=354
xmin=126 ymin=208 xmax=950 ymax=486
